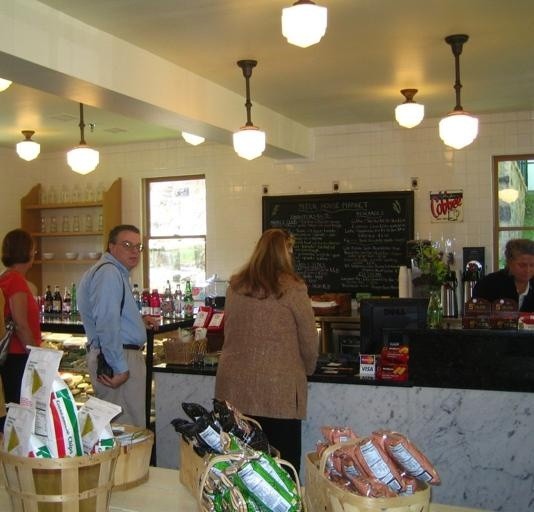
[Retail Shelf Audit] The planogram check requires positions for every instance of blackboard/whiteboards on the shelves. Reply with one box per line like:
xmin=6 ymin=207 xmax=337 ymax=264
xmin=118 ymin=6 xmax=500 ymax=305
xmin=261 ymin=191 xmax=414 ymax=297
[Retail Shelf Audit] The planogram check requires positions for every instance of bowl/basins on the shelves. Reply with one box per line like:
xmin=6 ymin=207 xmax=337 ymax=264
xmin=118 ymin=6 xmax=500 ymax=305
xmin=41 ymin=252 xmax=102 ymax=260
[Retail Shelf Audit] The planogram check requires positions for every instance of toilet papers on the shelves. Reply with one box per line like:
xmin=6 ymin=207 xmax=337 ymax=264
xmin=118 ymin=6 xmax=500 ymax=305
xmin=398 ymin=265 xmax=409 ymax=299
xmin=408 ymin=269 xmax=414 ymax=300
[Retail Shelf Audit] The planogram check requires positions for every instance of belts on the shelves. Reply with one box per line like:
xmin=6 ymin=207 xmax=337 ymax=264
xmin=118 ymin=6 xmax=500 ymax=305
xmin=86 ymin=344 xmax=145 ymax=350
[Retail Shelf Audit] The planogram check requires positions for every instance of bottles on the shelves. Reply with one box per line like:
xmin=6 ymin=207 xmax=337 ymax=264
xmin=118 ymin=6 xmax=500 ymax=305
xmin=132 ymin=280 xmax=194 ymax=318
xmin=40 ymin=215 xmax=103 ymax=233
xmin=41 ymin=182 xmax=103 ymax=204
xmin=37 ymin=283 xmax=77 ymax=315
xmin=426 ymin=291 xmax=440 ymax=330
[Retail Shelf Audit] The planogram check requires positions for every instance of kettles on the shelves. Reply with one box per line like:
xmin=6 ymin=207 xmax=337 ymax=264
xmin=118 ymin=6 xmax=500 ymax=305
xmin=464 ymin=261 xmax=482 ymax=303
xmin=441 ymin=271 xmax=458 ymax=318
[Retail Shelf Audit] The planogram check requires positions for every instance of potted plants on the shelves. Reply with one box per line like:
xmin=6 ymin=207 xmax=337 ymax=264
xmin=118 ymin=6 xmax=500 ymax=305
xmin=414 ymin=237 xmax=452 ymax=327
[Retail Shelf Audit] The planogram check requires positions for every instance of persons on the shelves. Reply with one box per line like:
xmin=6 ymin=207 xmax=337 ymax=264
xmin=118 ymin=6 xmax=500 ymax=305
xmin=76 ymin=225 xmax=147 ymax=428
xmin=213 ymin=227 xmax=319 ymax=479
xmin=0 ymin=228 xmax=41 ymax=404
xmin=472 ymin=239 xmax=533 ymax=311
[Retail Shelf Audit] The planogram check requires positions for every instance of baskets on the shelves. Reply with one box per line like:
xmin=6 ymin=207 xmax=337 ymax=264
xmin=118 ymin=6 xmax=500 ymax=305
xmin=163 ymin=338 xmax=208 ymax=366
xmin=179 ymin=414 xmax=302 ymax=512
xmin=304 ymin=439 xmax=431 ymax=512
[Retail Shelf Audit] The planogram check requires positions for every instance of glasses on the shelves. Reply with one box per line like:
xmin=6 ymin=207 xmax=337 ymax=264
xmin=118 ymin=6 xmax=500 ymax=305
xmin=117 ymin=242 xmax=144 ymax=253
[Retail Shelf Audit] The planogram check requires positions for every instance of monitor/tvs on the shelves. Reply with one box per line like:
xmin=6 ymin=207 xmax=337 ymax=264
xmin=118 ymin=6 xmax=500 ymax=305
xmin=360 ymin=297 xmax=428 ymax=354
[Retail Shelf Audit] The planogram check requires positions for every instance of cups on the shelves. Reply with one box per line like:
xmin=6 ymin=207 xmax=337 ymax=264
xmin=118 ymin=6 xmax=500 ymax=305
xmin=398 ymin=265 xmax=414 ymax=299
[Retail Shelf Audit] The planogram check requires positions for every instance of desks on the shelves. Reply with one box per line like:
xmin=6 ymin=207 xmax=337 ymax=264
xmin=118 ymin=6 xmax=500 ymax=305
xmin=0 ymin=432 xmax=490 ymax=511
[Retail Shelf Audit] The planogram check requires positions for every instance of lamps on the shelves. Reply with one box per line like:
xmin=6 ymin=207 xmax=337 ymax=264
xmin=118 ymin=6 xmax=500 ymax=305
xmin=498 ymin=188 xmax=519 ymax=203
xmin=66 ymin=103 xmax=100 ymax=175
xmin=232 ymin=60 xmax=266 ymax=162
xmin=394 ymin=90 xmax=424 ymax=130
xmin=438 ymin=35 xmax=478 ymax=149
xmin=15 ymin=130 xmax=40 ymax=160
xmin=280 ymin=1 xmax=328 ymax=48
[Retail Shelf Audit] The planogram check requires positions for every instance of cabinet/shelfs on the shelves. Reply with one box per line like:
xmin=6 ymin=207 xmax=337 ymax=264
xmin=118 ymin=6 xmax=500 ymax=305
xmin=40 ymin=318 xmax=194 ymax=466
xmin=20 ymin=175 xmax=122 ymax=310
